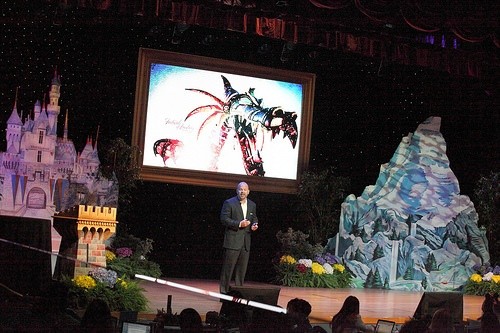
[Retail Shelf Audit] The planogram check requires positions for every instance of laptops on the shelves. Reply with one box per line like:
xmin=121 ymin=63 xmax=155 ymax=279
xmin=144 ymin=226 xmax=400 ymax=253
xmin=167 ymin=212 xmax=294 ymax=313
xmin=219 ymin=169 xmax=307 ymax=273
xmin=374 ymin=319 xmax=395 ymax=333
xmin=121 ymin=321 xmax=152 ymax=333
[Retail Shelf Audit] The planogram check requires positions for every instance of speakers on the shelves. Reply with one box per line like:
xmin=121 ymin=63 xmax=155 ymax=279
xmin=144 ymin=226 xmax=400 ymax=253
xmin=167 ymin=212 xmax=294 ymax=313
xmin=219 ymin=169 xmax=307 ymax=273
xmin=219 ymin=286 xmax=283 ymax=319
xmin=413 ymin=292 xmax=463 ymax=320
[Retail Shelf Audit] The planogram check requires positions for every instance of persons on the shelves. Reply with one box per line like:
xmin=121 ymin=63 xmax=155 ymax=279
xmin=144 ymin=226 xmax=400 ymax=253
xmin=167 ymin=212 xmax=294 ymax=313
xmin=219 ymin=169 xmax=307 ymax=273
xmin=331 ymin=293 xmax=500 ymax=333
xmin=178 ymin=298 xmax=328 ymax=333
xmin=217 ymin=182 xmax=258 ymax=302
xmin=74 ymin=299 xmax=119 ymax=333
xmin=31 ymin=282 xmax=69 ymax=314
